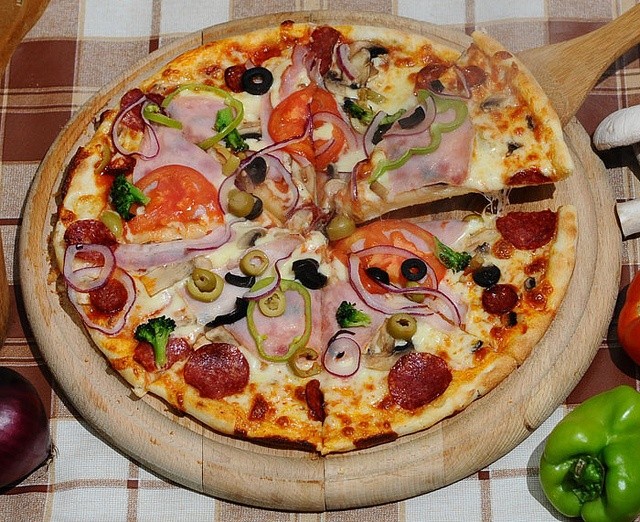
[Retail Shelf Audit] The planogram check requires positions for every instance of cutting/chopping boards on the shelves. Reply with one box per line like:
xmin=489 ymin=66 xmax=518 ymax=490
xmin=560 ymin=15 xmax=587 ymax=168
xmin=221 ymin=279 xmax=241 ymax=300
xmin=15 ymin=2 xmax=640 ymax=515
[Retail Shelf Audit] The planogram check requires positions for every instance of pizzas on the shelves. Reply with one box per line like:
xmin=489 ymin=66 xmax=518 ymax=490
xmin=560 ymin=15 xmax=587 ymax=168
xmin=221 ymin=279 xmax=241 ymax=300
xmin=53 ymin=22 xmax=579 ymax=456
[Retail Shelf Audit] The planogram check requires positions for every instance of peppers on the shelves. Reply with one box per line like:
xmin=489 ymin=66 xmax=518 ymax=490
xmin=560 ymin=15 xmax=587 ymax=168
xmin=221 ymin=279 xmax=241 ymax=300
xmin=539 ymin=384 xmax=640 ymax=522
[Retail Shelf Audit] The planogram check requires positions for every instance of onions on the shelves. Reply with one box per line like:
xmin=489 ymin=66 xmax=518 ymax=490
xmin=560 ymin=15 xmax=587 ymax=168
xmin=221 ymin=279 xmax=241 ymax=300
xmin=0 ymin=366 xmax=58 ymax=491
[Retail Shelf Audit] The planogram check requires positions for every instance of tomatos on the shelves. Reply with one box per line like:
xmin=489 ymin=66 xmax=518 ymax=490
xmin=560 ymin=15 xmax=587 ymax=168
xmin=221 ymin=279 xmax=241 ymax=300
xmin=617 ymin=266 xmax=640 ymax=366
